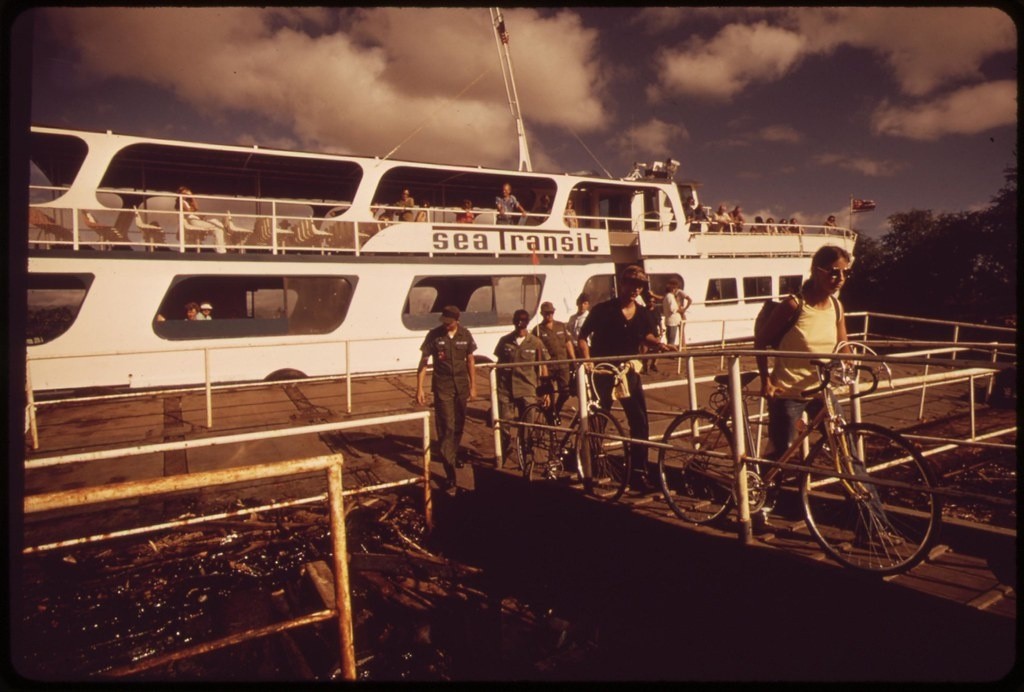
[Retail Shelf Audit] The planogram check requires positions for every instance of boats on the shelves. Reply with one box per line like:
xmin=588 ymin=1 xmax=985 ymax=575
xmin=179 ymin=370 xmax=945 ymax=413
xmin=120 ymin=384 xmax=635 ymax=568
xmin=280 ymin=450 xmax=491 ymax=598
xmin=24 ymin=6 xmax=859 ymax=408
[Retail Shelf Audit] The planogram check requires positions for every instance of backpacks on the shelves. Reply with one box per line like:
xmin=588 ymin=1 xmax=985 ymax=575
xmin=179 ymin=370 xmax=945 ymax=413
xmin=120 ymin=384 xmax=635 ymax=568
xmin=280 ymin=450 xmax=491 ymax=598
xmin=753 ymin=295 xmax=840 ymax=349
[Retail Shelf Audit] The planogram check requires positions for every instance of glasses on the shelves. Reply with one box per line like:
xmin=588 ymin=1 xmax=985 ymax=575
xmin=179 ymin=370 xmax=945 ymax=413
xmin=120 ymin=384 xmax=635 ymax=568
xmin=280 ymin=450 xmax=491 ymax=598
xmin=819 ymin=266 xmax=853 ymax=280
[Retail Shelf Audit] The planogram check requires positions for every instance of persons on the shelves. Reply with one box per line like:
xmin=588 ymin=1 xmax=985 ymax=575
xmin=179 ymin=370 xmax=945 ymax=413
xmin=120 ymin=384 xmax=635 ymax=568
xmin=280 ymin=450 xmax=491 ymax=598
xmin=492 ymin=310 xmax=550 ymax=469
xmin=564 ymin=200 xmax=578 ymax=228
xmin=755 ymin=246 xmax=889 ymax=545
xmin=175 ymin=187 xmax=227 ymax=254
xmin=185 ymin=303 xmax=200 ymax=321
xmin=456 ymin=199 xmax=480 ymax=224
xmin=415 ymin=201 xmax=430 ymax=222
xmin=682 ymin=197 xmax=836 ymax=235
xmin=370 ymin=203 xmax=379 ymax=216
xmin=378 ymin=204 xmax=395 ymax=228
xmin=417 ymin=305 xmax=478 ymax=491
xmin=567 ymin=295 xmax=595 ymax=356
xmin=537 ymin=194 xmax=552 ymax=223
xmin=633 ymin=279 xmax=693 ymax=375
xmin=395 ymin=188 xmax=414 ymax=222
xmin=577 ymin=266 xmax=677 ymax=495
xmin=196 ymin=303 xmax=212 ymax=321
xmin=495 ymin=184 xmax=525 ymax=226
xmin=530 ymin=301 xmax=577 ymax=427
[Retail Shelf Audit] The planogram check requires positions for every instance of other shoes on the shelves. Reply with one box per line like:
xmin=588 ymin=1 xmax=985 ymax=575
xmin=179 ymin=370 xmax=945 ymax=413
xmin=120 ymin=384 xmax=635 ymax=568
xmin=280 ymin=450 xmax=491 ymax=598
xmin=630 ymin=473 xmax=656 ymax=492
xmin=446 ymin=476 xmax=456 ymax=487
xmin=556 ymin=413 xmax=562 ymax=426
xmin=857 ymin=530 xmax=905 ymax=546
xmin=456 ymin=458 xmax=463 ymax=468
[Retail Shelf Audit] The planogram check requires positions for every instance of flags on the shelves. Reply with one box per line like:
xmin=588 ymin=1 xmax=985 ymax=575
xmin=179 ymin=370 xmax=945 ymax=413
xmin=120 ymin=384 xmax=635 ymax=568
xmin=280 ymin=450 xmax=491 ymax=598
xmin=850 ymin=198 xmax=877 ymax=213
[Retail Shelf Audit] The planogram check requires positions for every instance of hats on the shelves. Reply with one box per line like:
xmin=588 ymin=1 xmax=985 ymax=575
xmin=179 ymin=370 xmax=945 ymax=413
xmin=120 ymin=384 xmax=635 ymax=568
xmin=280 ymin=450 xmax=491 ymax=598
xmin=540 ymin=302 xmax=555 ymax=312
xmin=200 ymin=303 xmax=213 ymax=309
xmin=622 ymin=265 xmax=649 ymax=284
xmin=439 ymin=306 xmax=461 ymax=325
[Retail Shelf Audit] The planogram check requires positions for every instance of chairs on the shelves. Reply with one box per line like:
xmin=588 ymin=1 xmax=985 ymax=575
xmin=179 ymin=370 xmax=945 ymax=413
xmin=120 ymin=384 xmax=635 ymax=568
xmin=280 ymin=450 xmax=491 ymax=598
xmin=26 ymin=206 xmax=373 ymax=254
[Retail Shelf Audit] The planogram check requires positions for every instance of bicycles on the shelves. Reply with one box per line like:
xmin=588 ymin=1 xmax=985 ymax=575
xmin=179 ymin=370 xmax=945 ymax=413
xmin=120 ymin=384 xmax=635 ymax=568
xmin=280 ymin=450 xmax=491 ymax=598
xmin=657 ymin=359 xmax=944 ymax=578
xmin=515 ymin=360 xmax=647 ymax=505
